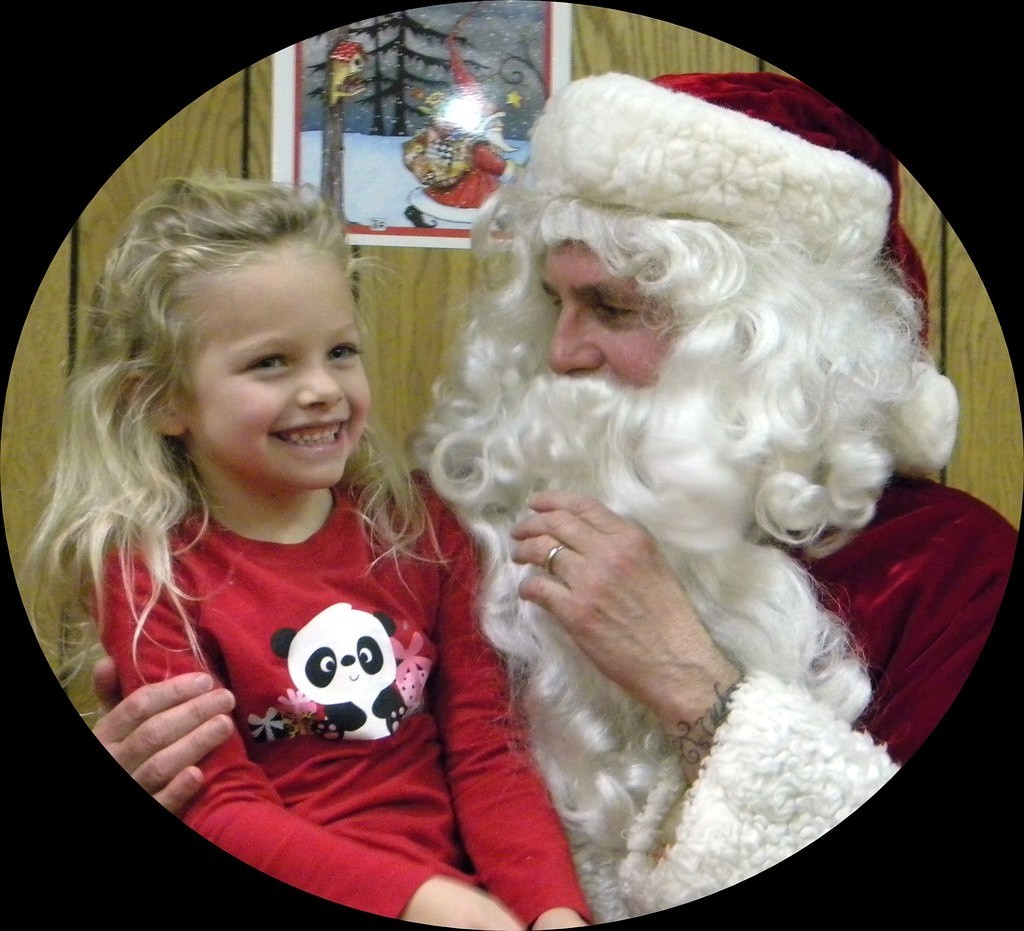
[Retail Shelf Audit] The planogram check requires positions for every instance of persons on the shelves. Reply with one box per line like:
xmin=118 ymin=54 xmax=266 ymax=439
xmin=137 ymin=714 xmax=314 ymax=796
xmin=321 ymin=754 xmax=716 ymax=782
xmin=24 ymin=165 xmax=591 ymax=931
xmin=414 ymin=67 xmax=1021 ymax=922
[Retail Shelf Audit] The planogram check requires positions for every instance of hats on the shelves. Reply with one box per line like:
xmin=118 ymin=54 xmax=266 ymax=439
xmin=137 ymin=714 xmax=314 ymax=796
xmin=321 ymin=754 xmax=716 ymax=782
xmin=533 ymin=68 xmax=901 ymax=269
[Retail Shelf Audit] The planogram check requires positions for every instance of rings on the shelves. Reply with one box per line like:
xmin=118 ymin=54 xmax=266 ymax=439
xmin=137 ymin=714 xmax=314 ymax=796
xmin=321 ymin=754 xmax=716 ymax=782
xmin=545 ymin=543 xmax=569 ymax=576
